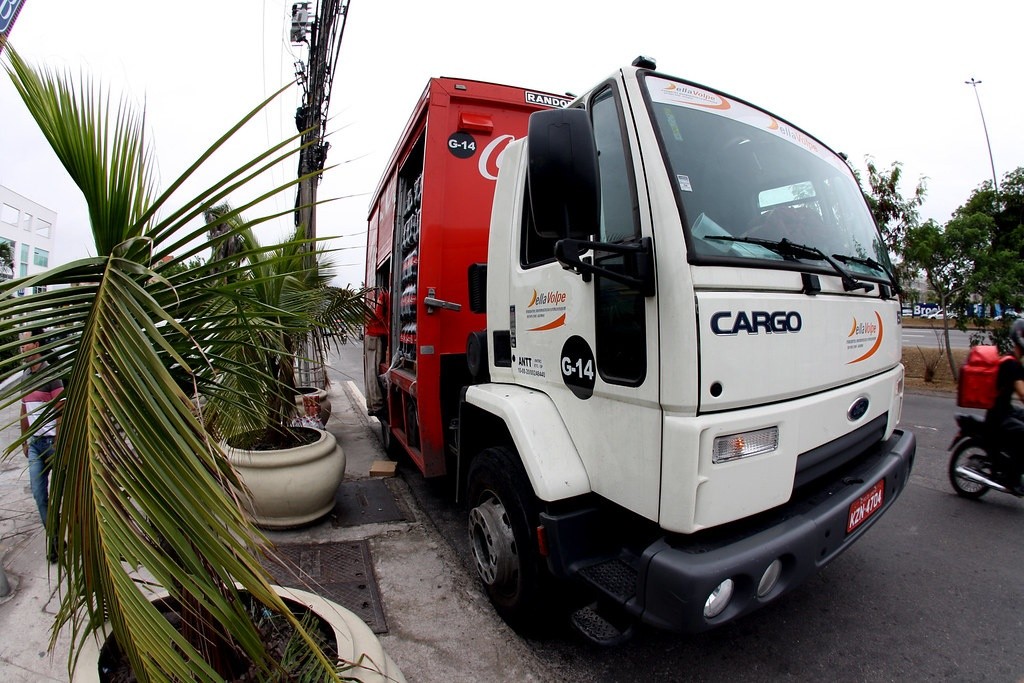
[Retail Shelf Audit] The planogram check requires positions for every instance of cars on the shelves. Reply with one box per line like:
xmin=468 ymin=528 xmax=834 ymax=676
xmin=322 ymin=329 xmax=331 ymax=338
xmin=994 ymin=314 xmax=1003 ymax=321
xmin=930 ymin=310 xmax=959 ymax=320
xmin=921 ymin=311 xmax=938 ymax=318
xmin=901 ymin=309 xmax=912 ymax=316
xmin=359 ymin=326 xmax=364 ymax=339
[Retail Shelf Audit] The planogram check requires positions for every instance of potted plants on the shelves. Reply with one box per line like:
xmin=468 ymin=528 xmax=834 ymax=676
xmin=0 ymin=33 xmax=408 ymax=683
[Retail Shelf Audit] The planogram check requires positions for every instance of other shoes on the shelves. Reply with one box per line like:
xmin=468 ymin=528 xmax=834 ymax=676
xmin=46 ymin=535 xmax=68 ymax=563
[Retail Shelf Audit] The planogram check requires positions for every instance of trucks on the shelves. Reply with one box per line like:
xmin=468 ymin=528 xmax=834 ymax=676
xmin=360 ymin=56 xmax=918 ymax=645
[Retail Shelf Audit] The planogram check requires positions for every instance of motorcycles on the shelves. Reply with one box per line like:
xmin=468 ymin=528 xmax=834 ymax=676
xmin=341 ymin=334 xmax=347 ymax=344
xmin=945 ymin=413 xmax=1024 ymax=499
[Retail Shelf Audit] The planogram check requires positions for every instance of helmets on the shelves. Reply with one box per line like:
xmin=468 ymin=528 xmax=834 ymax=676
xmin=1010 ymin=318 xmax=1024 ymax=356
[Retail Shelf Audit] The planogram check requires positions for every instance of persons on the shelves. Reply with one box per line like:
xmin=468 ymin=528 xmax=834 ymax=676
xmin=19 ymin=332 xmax=72 ymax=559
xmin=983 ymin=320 xmax=1024 ymax=497
